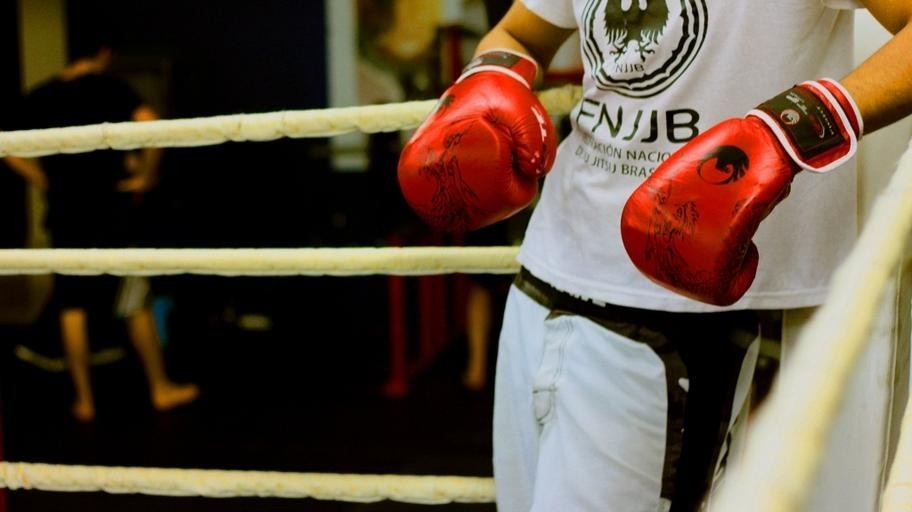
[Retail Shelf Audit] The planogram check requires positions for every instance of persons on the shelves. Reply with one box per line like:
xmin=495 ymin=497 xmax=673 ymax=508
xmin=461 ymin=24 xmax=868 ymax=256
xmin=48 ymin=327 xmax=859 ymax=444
xmin=397 ymin=0 xmax=912 ymax=509
xmin=1 ymin=24 xmax=195 ymax=419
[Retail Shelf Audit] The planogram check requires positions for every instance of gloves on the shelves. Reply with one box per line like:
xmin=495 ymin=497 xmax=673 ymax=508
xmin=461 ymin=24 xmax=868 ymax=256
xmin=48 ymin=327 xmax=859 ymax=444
xmin=397 ymin=49 xmax=557 ymax=234
xmin=620 ymin=78 xmax=865 ymax=305
